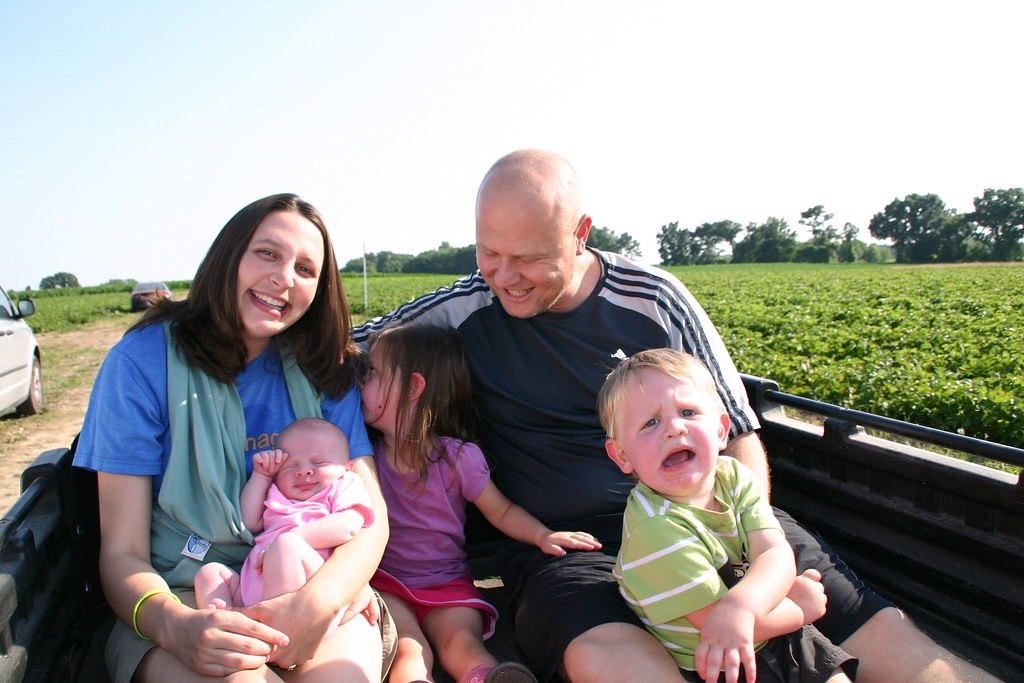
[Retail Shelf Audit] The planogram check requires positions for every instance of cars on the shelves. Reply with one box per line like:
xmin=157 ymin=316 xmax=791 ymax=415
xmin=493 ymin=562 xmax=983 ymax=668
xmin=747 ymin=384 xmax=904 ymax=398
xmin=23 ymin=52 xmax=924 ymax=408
xmin=0 ymin=288 xmax=44 ymax=418
xmin=131 ymin=282 xmax=170 ymax=312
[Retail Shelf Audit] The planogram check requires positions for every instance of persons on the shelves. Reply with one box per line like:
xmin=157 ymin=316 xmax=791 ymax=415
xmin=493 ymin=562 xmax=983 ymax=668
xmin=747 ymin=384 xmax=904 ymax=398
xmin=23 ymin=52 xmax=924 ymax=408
xmin=72 ymin=193 xmax=398 ymax=683
xmin=347 ymin=149 xmax=1005 ymax=683
xmin=360 ymin=326 xmax=604 ymax=683
xmin=597 ymin=347 xmax=860 ymax=683
xmin=194 ymin=419 xmax=376 ymax=611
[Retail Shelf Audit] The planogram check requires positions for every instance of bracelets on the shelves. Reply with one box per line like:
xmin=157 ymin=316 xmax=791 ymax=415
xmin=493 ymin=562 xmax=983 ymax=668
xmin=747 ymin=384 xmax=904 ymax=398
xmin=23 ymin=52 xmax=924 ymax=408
xmin=133 ymin=589 xmax=181 ymax=641
